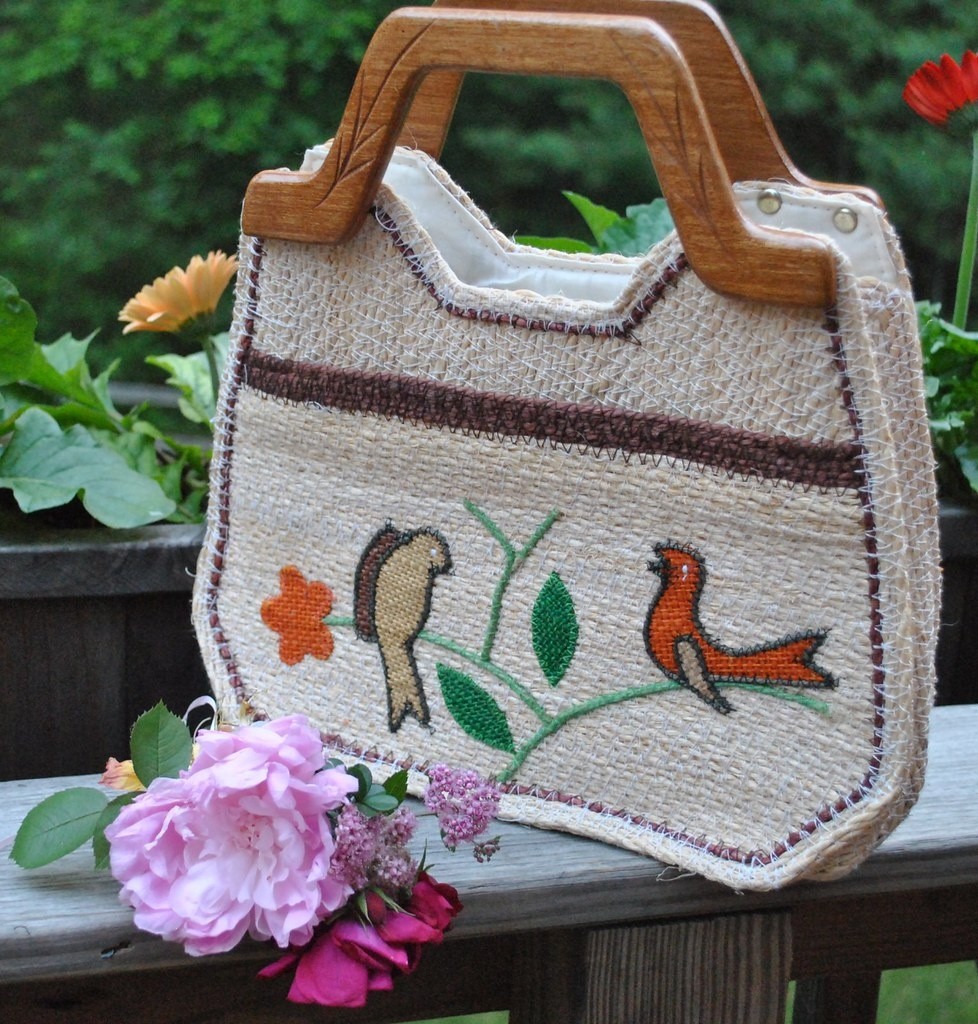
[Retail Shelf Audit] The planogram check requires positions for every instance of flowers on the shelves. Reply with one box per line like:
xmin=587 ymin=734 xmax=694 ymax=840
xmin=903 ymin=47 xmax=978 ymax=329
xmin=112 ymin=247 xmax=239 ymax=402
xmin=8 ymin=693 xmax=510 ymax=1007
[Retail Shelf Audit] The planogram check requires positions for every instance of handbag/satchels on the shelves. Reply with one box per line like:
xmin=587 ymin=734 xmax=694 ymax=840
xmin=191 ymin=0 xmax=943 ymax=894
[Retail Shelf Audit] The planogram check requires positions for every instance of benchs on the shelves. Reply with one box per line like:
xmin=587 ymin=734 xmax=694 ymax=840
xmin=0 ymin=703 xmax=978 ymax=1024
